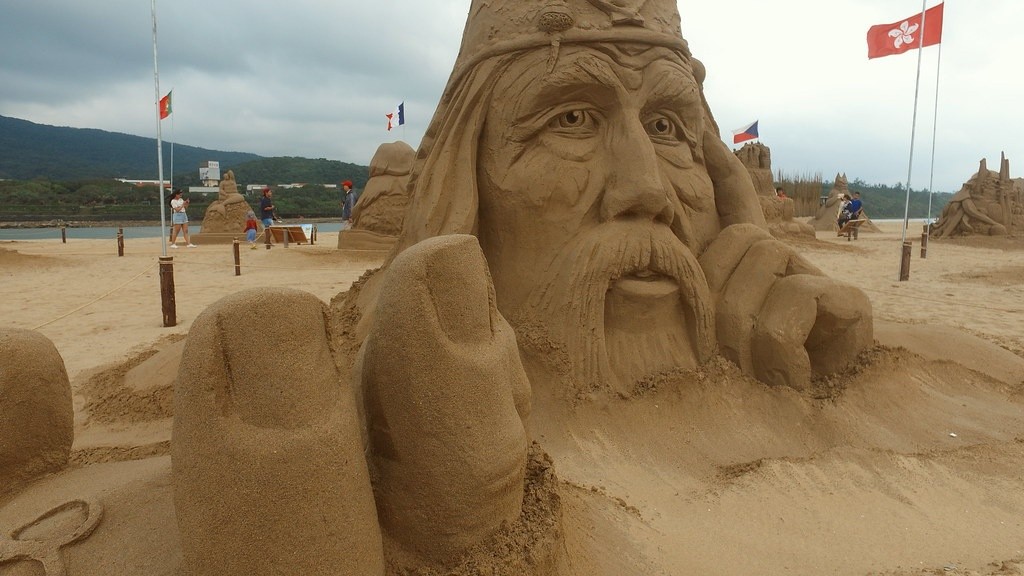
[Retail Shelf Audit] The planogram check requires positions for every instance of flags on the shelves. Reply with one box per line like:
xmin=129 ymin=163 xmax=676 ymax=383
xmin=156 ymin=91 xmax=173 ymax=119
xmin=732 ymin=122 xmax=758 ymax=144
xmin=386 ymin=103 xmax=405 ymax=131
xmin=867 ymin=2 xmax=944 ymax=59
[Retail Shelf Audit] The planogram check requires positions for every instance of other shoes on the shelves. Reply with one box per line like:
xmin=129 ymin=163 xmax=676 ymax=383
xmin=187 ymin=243 xmax=197 ymax=248
xmin=170 ymin=243 xmax=178 ymax=249
xmin=251 ymin=245 xmax=257 ymax=249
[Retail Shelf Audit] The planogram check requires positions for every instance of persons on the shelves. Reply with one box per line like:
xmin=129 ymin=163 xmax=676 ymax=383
xmin=164 ymin=189 xmax=197 ymax=248
xmin=341 ymin=180 xmax=357 ymax=224
xmin=776 ymin=187 xmax=786 ymax=198
xmin=243 ymin=211 xmax=258 ymax=250
xmin=0 ymin=0 xmax=872 ymax=576
xmin=836 ymin=192 xmax=863 ymax=230
xmin=261 ymin=188 xmax=278 ymax=227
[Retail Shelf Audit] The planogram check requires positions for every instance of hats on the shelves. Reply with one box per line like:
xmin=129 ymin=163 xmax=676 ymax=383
xmin=247 ymin=211 xmax=255 ymax=219
xmin=341 ymin=180 xmax=352 ymax=188
xmin=263 ymin=187 xmax=270 ymax=195
